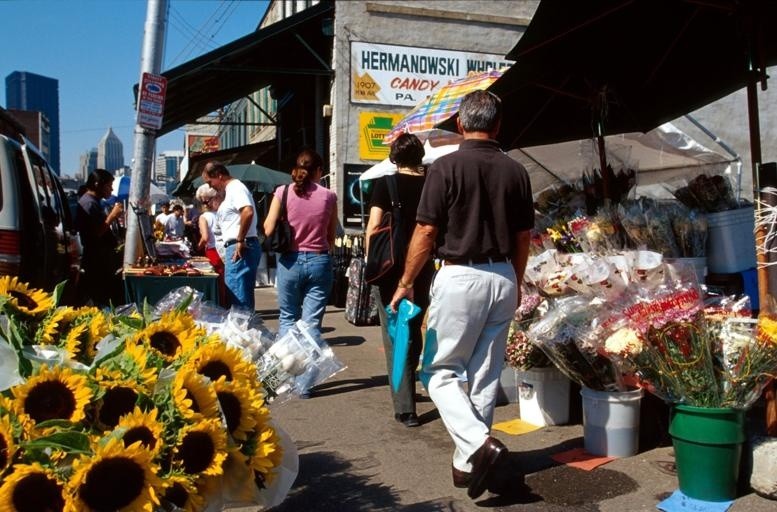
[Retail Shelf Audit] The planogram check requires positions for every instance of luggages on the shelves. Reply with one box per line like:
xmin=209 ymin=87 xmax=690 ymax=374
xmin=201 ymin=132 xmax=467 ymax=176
xmin=344 ymin=256 xmax=380 ymax=326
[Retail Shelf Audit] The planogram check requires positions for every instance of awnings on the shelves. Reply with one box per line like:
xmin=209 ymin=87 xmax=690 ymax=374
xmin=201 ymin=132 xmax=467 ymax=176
xmin=173 ymin=137 xmax=277 ymax=200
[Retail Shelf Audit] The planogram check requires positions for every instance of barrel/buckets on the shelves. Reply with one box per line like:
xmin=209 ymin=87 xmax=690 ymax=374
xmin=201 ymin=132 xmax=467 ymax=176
xmin=579 ymin=385 xmax=644 ymax=459
xmin=667 ymin=403 xmax=746 ymax=503
xmin=514 ymin=365 xmax=571 ymax=427
xmin=703 ymin=201 xmax=758 ymax=274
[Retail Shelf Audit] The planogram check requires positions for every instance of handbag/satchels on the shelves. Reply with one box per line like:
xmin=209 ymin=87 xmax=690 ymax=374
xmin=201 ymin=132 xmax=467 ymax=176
xmin=364 ymin=211 xmax=404 ymax=287
xmin=262 ymin=218 xmax=293 ymax=253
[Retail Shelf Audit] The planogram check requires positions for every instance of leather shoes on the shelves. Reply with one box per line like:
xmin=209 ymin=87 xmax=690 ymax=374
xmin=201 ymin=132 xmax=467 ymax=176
xmin=395 ymin=412 xmax=419 ymax=427
xmin=452 ymin=463 xmax=471 ymax=487
xmin=468 ymin=437 xmax=508 ymax=499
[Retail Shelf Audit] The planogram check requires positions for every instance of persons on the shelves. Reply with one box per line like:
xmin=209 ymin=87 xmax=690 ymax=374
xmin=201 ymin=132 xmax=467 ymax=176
xmin=365 ymin=134 xmax=445 ymax=426
xmin=263 ymin=149 xmax=338 ymax=398
xmin=61 ymin=160 xmax=262 ymax=315
xmin=391 ymin=89 xmax=534 ymax=494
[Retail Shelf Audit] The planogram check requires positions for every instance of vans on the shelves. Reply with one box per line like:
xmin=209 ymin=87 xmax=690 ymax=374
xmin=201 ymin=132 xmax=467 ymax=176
xmin=0 ymin=132 xmax=68 ymax=289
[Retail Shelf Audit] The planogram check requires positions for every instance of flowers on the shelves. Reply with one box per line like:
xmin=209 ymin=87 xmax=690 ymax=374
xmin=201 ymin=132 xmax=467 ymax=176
xmin=0 ymin=271 xmax=317 ymax=511
xmin=502 ymin=157 xmax=777 ymax=411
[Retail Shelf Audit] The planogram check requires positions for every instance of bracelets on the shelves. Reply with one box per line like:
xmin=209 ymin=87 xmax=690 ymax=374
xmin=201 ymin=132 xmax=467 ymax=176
xmin=237 ymin=239 xmax=244 ymax=242
xmin=398 ymin=280 xmax=414 ymax=288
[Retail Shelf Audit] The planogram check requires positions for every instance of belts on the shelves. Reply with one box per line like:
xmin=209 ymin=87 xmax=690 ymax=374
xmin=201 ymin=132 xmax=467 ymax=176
xmin=224 ymin=237 xmax=257 ymax=248
xmin=444 ymin=255 xmax=506 ymax=264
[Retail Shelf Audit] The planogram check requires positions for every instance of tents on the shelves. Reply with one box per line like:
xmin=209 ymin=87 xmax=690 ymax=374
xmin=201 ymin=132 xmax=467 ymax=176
xmin=359 ymin=126 xmax=463 ymax=230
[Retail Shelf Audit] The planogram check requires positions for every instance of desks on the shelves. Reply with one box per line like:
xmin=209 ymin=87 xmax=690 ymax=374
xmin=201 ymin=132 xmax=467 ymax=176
xmin=123 ymin=255 xmax=221 ymax=305
xmin=154 ymin=236 xmax=186 ymax=257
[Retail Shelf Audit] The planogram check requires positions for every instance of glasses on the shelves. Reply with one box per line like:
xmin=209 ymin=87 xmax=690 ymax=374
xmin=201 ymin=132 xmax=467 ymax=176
xmin=203 ymin=197 xmax=213 ymax=204
